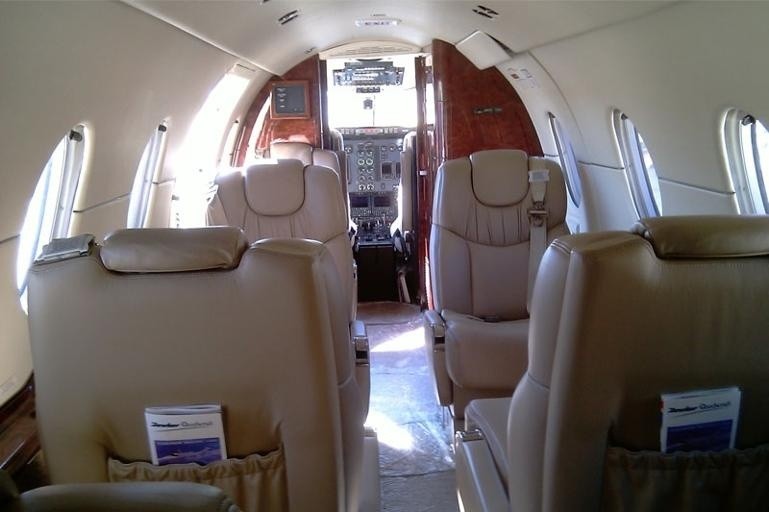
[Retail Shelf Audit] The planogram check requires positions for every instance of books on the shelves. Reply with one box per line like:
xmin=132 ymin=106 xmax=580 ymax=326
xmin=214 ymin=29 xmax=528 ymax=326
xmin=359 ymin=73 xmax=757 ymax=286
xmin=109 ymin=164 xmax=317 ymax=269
xmin=143 ymin=402 xmax=228 ymax=465
xmin=656 ymin=384 xmax=743 ymax=454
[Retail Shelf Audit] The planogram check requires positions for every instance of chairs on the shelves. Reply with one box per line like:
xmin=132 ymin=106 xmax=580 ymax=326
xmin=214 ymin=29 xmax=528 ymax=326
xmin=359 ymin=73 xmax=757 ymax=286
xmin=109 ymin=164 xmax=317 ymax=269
xmin=20 ymin=129 xmax=383 ymax=512
xmin=454 ymin=216 xmax=768 ymax=512
xmin=389 ymin=131 xmax=435 ymax=304
xmin=422 ymin=147 xmax=572 ymax=418
